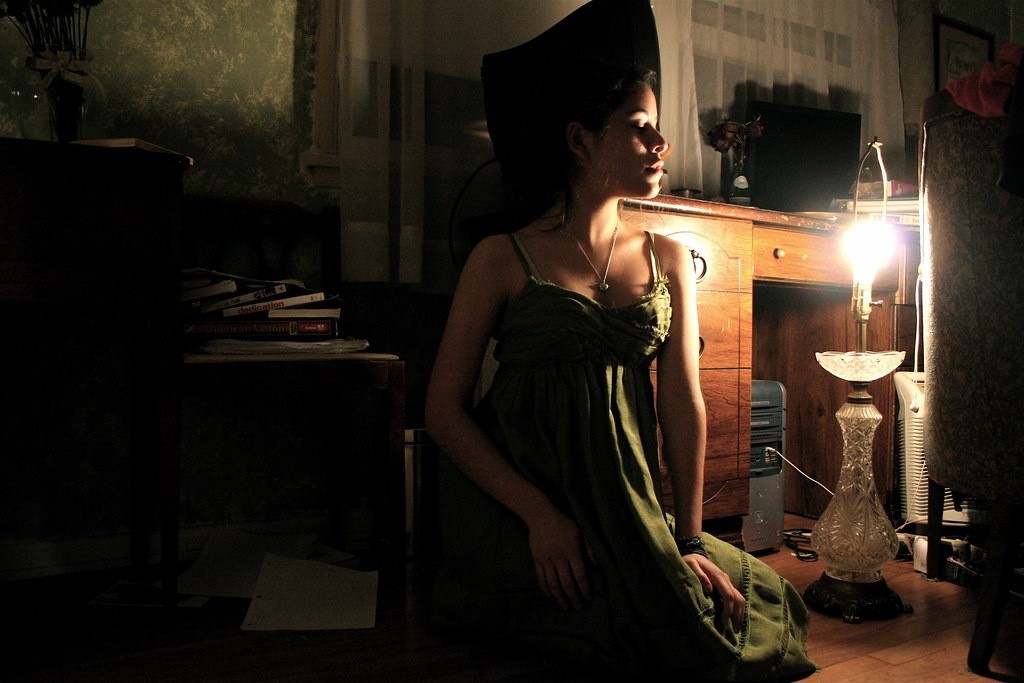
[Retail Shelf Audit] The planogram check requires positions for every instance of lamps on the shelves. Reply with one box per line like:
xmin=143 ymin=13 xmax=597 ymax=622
xmin=801 ymin=135 xmax=911 ymax=625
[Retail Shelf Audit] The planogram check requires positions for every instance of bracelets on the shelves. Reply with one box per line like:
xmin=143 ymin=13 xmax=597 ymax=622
xmin=676 ymin=534 xmax=709 ymax=559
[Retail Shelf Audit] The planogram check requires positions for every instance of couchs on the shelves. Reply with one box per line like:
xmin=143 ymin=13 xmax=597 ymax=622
xmin=921 ymin=44 xmax=1024 ymax=674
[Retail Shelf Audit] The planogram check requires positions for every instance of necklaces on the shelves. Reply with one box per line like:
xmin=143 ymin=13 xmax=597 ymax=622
xmin=561 ymin=208 xmax=620 ymax=292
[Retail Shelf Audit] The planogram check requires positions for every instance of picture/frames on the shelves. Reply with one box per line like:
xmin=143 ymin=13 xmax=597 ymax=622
xmin=933 ymin=14 xmax=995 ymax=93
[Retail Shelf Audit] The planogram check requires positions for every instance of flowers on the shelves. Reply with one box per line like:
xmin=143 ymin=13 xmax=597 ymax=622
xmin=705 ymin=115 xmax=766 ymax=153
xmin=0 ymin=0 xmax=112 ymax=142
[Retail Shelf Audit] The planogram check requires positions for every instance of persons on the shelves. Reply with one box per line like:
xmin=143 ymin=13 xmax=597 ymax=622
xmin=421 ymin=56 xmax=817 ymax=683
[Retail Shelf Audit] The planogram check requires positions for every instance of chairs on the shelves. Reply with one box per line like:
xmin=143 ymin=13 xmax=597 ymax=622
xmin=123 ymin=196 xmax=408 ymax=612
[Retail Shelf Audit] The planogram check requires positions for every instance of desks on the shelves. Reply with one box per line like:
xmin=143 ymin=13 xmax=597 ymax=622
xmin=0 ymin=138 xmax=186 ymax=602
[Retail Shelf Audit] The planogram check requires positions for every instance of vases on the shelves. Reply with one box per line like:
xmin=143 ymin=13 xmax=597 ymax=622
xmin=26 ymin=42 xmax=95 ymax=141
xmin=726 ymin=148 xmax=750 ymax=206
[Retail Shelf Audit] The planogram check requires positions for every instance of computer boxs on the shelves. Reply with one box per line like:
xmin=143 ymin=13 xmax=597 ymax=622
xmin=741 ymin=381 xmax=785 ymax=554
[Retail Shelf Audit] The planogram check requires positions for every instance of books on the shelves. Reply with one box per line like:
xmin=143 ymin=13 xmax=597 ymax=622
xmin=173 ymin=268 xmax=371 ymax=353
xmin=68 ymin=137 xmax=194 ymax=166
xmin=179 ymin=524 xmax=379 ymax=632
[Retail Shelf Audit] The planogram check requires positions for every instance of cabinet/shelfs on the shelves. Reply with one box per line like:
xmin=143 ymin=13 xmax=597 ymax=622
xmin=623 ymin=194 xmax=922 ymax=551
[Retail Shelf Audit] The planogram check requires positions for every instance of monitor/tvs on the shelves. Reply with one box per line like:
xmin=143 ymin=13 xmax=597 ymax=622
xmin=742 ymin=101 xmax=862 ymax=216
